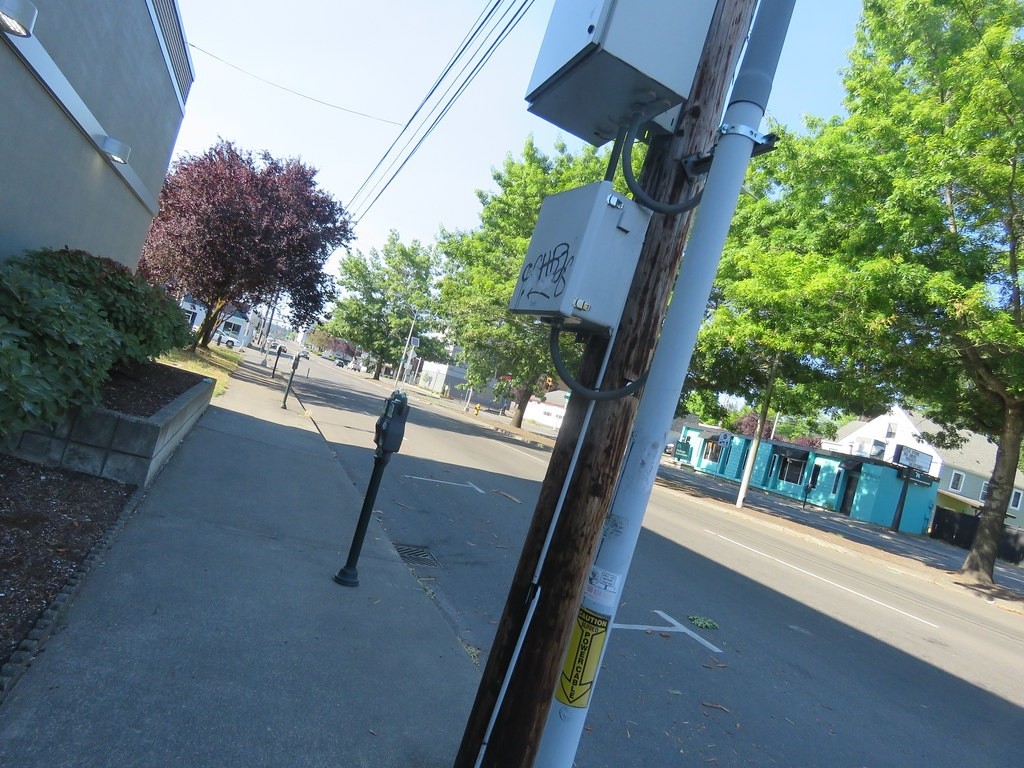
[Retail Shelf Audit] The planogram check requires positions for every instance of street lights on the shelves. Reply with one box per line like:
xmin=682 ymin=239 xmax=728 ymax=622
xmin=393 ymin=309 xmax=432 ymax=389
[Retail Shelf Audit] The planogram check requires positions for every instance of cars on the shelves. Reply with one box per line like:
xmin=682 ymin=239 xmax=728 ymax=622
xmin=331 ymin=360 xmax=345 ymax=368
xmin=270 ymin=343 xmax=279 ymax=349
xmin=300 ymin=350 xmax=310 ymax=359
xmin=281 ymin=345 xmax=287 ymax=353
xmin=192 ymin=325 xmax=241 ymax=348
xmin=346 ymin=360 xmax=361 ymax=372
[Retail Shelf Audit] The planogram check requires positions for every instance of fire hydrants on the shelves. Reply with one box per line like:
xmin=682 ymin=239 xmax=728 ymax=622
xmin=474 ymin=403 xmax=481 ymax=416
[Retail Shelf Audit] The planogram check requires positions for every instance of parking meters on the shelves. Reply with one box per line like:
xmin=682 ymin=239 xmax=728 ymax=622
xmin=330 ymin=389 xmax=411 ymax=589
xmin=280 ymin=352 xmax=303 ymax=410
xmin=270 ymin=345 xmax=283 ymax=379
